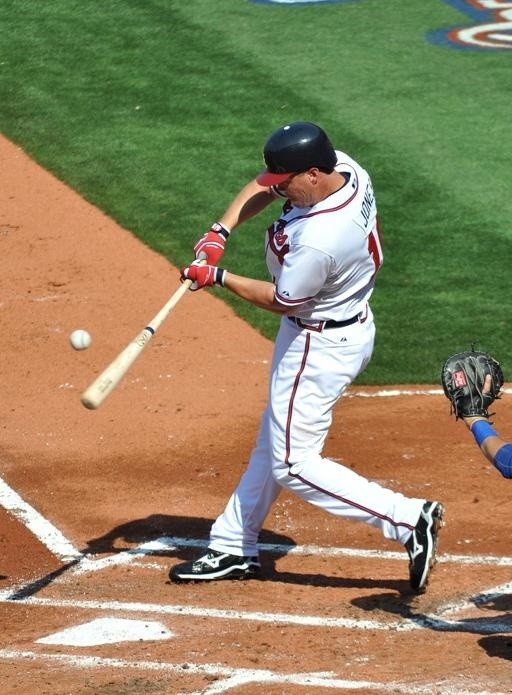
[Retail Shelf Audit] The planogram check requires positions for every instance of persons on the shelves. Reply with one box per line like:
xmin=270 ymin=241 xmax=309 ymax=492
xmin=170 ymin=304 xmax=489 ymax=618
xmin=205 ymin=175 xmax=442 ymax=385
xmin=439 ymin=348 xmax=511 ymax=481
xmin=166 ymin=116 xmax=445 ymax=598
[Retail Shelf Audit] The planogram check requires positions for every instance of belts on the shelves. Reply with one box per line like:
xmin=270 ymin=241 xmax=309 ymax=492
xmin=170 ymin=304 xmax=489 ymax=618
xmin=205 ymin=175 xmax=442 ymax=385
xmin=288 ymin=311 xmax=367 ymax=328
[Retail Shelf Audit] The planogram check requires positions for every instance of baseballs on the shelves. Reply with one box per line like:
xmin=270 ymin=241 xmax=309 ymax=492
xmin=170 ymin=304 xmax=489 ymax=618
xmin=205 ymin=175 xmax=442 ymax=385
xmin=69 ymin=329 xmax=90 ymax=350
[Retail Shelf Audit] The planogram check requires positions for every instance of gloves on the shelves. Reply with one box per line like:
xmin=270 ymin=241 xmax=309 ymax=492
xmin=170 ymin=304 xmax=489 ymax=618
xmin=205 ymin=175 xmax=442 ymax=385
xmin=194 ymin=221 xmax=230 ymax=266
xmin=179 ymin=259 xmax=227 ymax=291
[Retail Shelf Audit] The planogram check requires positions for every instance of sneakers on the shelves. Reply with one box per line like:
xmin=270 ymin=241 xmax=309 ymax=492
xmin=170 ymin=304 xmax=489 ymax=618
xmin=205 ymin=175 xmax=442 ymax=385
xmin=405 ymin=502 xmax=444 ymax=591
xmin=168 ymin=550 xmax=263 ymax=581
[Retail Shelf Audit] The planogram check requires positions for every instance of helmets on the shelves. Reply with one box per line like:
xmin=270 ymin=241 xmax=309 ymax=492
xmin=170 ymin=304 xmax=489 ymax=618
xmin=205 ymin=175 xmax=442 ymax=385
xmin=256 ymin=121 xmax=337 ymax=186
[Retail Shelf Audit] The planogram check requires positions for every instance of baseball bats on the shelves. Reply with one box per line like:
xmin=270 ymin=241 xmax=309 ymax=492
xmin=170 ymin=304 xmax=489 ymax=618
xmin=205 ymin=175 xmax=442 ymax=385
xmin=81 ymin=259 xmax=207 ymax=410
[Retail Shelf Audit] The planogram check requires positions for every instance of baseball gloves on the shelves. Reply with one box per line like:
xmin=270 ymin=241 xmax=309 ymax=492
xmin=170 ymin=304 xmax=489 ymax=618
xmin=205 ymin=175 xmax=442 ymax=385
xmin=442 ymin=343 xmax=505 ymax=418
